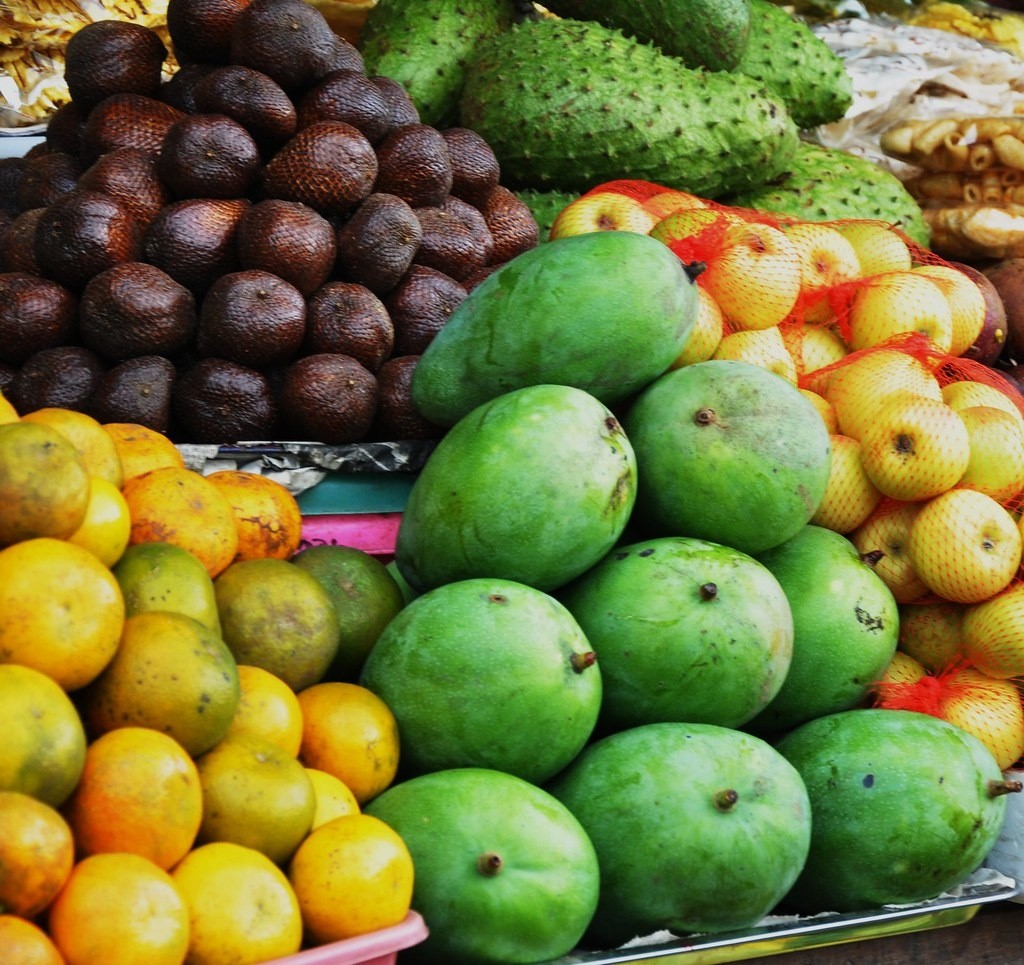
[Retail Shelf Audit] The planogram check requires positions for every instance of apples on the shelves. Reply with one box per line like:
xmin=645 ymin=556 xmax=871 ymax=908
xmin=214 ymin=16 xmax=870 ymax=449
xmin=557 ymin=193 xmax=1024 ymax=772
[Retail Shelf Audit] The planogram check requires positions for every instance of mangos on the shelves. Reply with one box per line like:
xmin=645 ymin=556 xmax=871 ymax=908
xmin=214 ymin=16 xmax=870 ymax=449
xmin=361 ymin=230 xmax=1009 ymax=965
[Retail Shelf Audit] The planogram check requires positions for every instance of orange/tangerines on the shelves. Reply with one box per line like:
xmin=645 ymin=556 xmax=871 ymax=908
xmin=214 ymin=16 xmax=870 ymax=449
xmin=1 ymin=394 xmax=415 ymax=964
